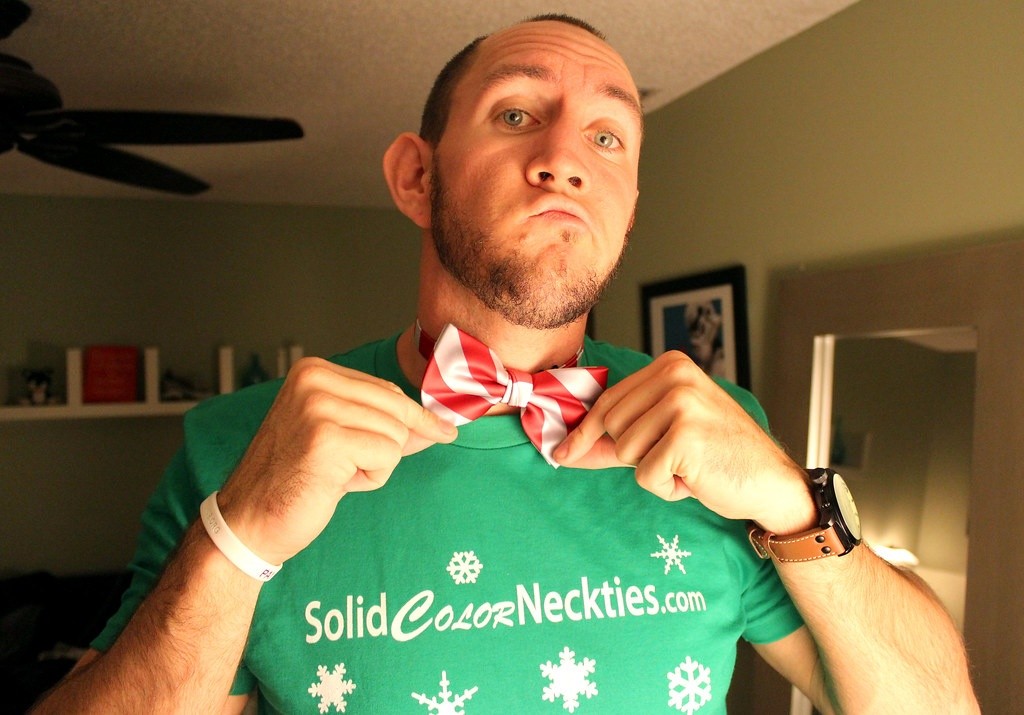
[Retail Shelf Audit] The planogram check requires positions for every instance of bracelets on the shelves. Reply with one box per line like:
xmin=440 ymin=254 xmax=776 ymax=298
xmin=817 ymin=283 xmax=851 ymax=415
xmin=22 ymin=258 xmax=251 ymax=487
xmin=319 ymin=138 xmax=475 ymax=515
xmin=200 ymin=490 xmax=282 ymax=582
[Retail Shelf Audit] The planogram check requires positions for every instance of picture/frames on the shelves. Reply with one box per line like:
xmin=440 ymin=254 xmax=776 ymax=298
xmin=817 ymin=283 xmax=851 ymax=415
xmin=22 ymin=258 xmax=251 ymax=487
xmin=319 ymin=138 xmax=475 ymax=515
xmin=638 ymin=263 xmax=753 ymax=392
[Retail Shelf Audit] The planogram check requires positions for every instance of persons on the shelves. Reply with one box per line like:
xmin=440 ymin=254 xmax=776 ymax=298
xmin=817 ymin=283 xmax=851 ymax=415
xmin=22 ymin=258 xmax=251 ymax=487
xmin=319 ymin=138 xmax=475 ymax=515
xmin=33 ymin=12 xmax=981 ymax=714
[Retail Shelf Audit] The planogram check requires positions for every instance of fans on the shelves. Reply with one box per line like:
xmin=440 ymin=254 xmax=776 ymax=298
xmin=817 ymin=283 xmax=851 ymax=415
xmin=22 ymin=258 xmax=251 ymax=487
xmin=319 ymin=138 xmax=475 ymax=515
xmin=0 ymin=0 xmax=305 ymax=197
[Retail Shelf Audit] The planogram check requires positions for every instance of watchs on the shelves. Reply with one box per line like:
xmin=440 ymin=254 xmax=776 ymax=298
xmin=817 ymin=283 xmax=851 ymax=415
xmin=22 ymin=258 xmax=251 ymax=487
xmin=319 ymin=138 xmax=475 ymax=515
xmin=745 ymin=468 xmax=862 ymax=563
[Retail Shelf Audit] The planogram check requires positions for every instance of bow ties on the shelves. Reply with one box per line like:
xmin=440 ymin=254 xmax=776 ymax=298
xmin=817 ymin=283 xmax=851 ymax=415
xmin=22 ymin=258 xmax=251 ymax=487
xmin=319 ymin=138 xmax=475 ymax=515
xmin=414 ymin=318 xmax=609 ymax=470
